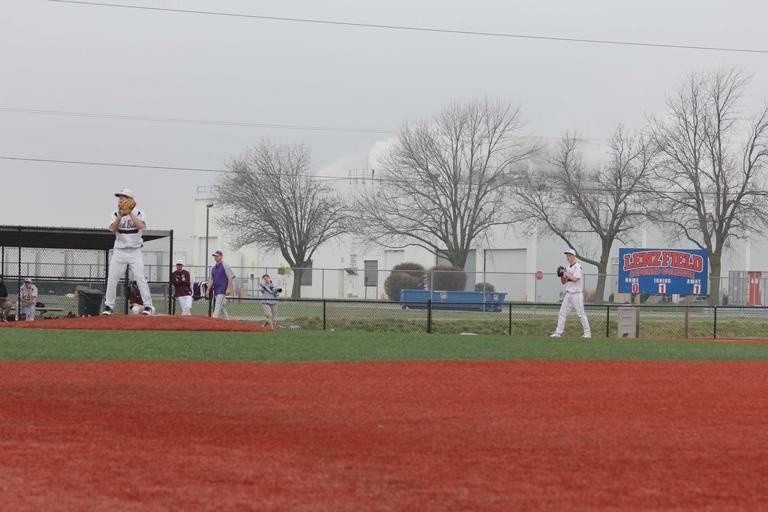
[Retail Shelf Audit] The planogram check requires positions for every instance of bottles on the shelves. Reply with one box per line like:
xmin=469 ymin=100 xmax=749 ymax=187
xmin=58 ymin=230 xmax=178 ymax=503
xmin=176 ymin=260 xmax=184 ymax=266
xmin=563 ymin=249 xmax=576 ymax=257
xmin=212 ymin=251 xmax=223 ymax=257
xmin=24 ymin=278 xmax=31 ymax=283
xmin=115 ymin=189 xmax=135 ymax=199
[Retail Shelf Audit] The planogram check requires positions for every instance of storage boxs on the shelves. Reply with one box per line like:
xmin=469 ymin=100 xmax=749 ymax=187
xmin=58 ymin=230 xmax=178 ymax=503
xmin=1 ymin=306 xmax=64 ymax=320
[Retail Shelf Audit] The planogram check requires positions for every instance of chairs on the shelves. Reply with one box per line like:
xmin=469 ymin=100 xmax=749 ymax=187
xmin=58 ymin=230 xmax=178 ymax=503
xmin=581 ymin=333 xmax=591 ymax=338
xmin=142 ymin=306 xmax=154 ymax=315
xmin=2 ymin=314 xmax=8 ymax=323
xmin=550 ymin=332 xmax=565 ymax=338
xmin=101 ymin=306 xmax=113 ymax=316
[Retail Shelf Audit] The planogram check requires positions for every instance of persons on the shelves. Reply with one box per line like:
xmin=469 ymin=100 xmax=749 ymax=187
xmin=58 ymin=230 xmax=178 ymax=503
xmin=19 ymin=277 xmax=40 ymax=321
xmin=0 ymin=274 xmax=13 ymax=323
xmin=205 ymin=250 xmax=236 ymax=320
xmin=257 ymin=273 xmax=283 ymax=329
xmin=130 ymin=280 xmax=145 ymax=314
xmin=172 ymin=259 xmax=194 ymax=317
xmin=101 ymin=189 xmax=156 ymax=317
xmin=550 ymin=249 xmax=593 ymax=338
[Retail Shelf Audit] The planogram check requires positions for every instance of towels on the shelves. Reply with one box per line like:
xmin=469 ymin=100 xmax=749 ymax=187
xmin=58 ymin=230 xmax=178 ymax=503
xmin=78 ymin=288 xmax=105 ymax=316
xmin=618 ymin=307 xmax=640 ymax=338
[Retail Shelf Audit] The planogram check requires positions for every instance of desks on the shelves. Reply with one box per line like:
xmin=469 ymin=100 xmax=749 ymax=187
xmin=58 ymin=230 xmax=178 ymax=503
xmin=205 ymin=201 xmax=214 ymax=279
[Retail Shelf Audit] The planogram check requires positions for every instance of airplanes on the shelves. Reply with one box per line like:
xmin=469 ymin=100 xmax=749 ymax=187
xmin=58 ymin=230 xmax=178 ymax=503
xmin=557 ymin=267 xmax=566 ymax=276
xmin=118 ymin=200 xmax=136 ymax=215
